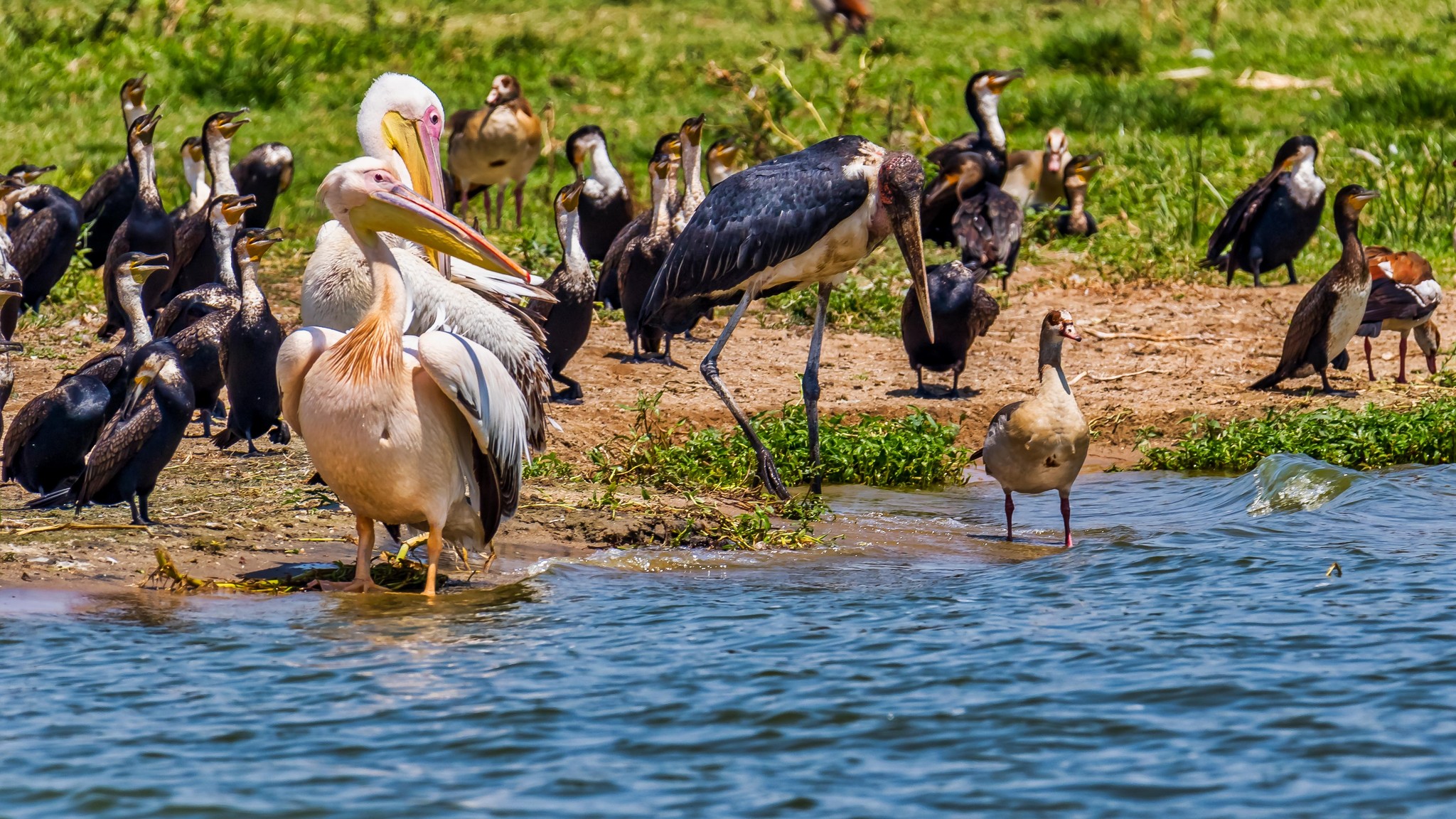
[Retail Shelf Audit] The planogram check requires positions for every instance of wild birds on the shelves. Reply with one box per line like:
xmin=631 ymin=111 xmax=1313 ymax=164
xmin=0 ymin=68 xmax=1103 ymax=615
xmin=983 ymin=308 xmax=1088 ymax=547
xmin=1197 ymin=134 xmax=1441 ymax=393
